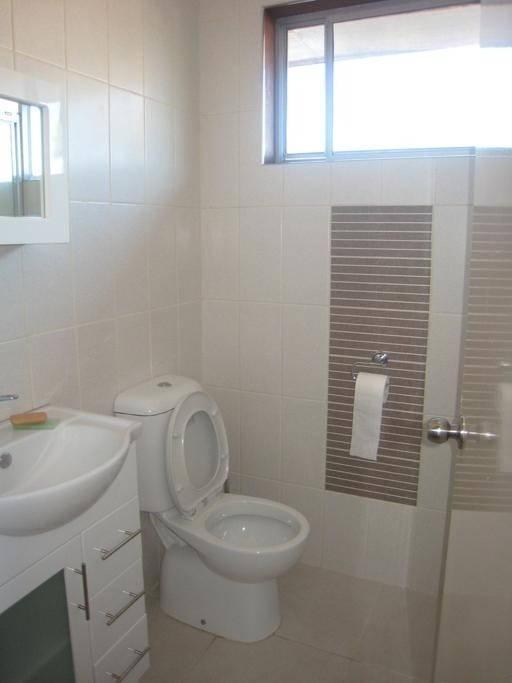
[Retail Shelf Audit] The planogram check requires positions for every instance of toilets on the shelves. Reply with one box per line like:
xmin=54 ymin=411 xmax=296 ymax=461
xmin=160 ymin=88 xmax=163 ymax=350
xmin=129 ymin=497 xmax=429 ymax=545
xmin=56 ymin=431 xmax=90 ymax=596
xmin=114 ymin=373 xmax=311 ymax=643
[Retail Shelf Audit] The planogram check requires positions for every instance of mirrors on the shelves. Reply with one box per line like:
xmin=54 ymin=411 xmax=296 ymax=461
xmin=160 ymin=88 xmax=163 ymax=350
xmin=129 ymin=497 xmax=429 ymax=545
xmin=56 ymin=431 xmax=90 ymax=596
xmin=0 ymin=95 xmax=46 ymax=217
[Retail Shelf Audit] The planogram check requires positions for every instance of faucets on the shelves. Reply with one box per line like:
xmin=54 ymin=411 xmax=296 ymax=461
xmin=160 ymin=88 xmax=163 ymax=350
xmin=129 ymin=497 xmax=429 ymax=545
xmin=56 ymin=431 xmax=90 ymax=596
xmin=0 ymin=393 xmax=19 ymax=402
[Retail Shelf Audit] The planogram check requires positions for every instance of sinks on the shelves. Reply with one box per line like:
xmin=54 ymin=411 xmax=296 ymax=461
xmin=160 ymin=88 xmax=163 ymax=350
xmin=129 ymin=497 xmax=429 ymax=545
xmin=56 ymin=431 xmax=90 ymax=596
xmin=0 ymin=405 xmax=144 ymax=539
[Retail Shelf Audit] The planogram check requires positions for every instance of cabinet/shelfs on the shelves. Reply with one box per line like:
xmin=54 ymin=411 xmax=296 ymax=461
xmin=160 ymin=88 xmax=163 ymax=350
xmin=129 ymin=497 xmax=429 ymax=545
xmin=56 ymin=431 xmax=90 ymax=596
xmin=0 ymin=405 xmax=152 ymax=683
